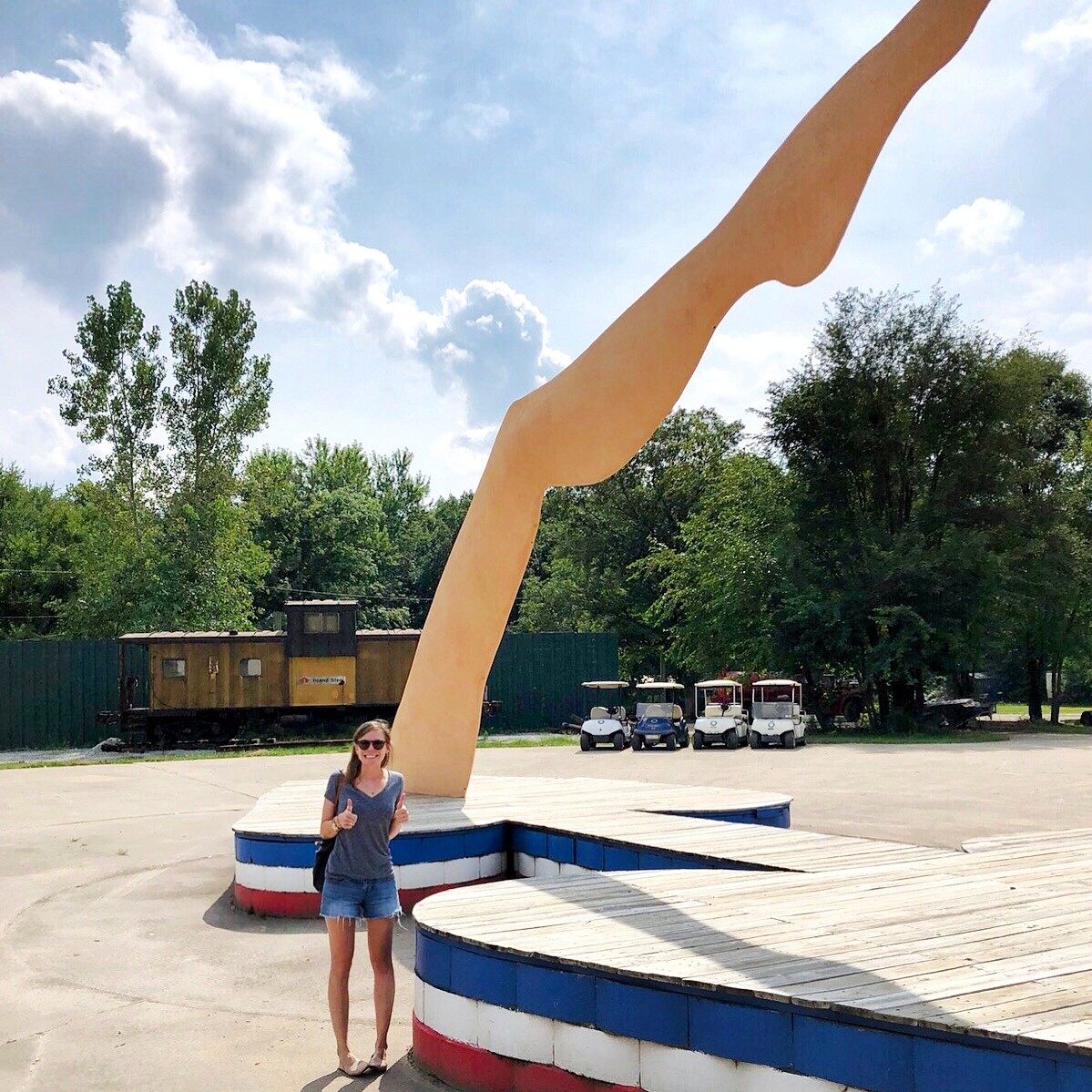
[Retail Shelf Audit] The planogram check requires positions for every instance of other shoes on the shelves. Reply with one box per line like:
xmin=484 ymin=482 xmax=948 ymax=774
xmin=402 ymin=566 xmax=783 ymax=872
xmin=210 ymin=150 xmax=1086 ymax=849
xmin=339 ymin=1056 xmax=371 ymax=1076
xmin=369 ymin=1053 xmax=387 ymax=1072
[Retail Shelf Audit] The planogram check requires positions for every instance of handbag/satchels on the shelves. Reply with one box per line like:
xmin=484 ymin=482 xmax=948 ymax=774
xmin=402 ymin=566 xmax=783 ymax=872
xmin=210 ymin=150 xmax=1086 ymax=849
xmin=313 ymin=772 xmax=346 ymax=892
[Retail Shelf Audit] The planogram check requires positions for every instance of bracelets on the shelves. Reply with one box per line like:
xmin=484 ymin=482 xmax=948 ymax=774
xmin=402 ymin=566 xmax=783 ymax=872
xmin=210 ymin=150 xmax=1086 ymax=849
xmin=335 ymin=816 xmax=342 ymax=829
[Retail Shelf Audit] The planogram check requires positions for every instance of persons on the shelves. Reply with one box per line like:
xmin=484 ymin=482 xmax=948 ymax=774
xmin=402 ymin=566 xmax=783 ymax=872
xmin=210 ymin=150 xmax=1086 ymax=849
xmin=320 ymin=720 xmax=409 ymax=1076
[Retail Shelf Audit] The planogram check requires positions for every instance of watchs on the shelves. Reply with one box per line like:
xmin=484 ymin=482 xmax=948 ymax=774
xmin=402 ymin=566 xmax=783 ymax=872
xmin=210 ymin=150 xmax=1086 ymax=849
xmin=331 ymin=817 xmax=341 ymax=831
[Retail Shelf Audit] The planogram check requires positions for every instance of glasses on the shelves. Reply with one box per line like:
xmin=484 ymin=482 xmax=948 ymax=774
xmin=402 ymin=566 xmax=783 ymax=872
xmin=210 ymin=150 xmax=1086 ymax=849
xmin=356 ymin=739 xmax=387 ymax=750
